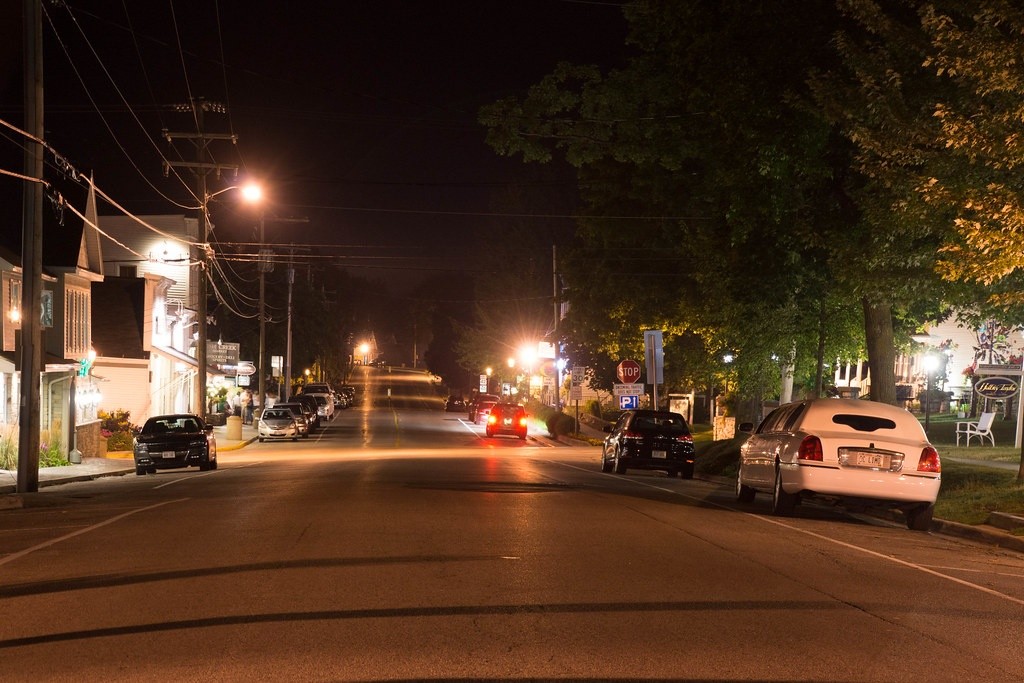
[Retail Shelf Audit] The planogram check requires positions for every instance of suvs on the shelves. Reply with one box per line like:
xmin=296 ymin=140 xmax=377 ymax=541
xmin=600 ymin=409 xmax=695 ymax=479
xmin=486 ymin=402 xmax=527 ymax=439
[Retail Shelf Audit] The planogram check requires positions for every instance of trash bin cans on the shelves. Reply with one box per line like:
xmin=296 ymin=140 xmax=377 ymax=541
xmin=226 ymin=416 xmax=242 ymax=440
xmin=253 ymin=409 xmax=259 ymax=429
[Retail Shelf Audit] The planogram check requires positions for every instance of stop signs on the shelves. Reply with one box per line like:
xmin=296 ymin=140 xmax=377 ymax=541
xmin=617 ymin=360 xmax=642 ymax=384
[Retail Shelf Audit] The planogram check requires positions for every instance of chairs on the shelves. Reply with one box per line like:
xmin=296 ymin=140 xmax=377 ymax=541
xmin=156 ymin=422 xmax=165 ymax=432
xmin=268 ymin=412 xmax=274 ymax=417
xmin=956 ymin=412 xmax=996 ymax=447
xmin=663 ymin=420 xmax=672 ymax=426
xmin=184 ymin=420 xmax=194 ymax=432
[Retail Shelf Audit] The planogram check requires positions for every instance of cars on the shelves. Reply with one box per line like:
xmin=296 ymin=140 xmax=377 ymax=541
xmin=273 ymin=382 xmax=356 ymax=438
xmin=131 ymin=414 xmax=217 ymax=475
xmin=444 ymin=394 xmax=466 ymax=412
xmin=467 ymin=393 xmax=501 ymax=425
xmin=255 ymin=408 xmax=298 ymax=442
xmin=736 ymin=399 xmax=942 ymax=531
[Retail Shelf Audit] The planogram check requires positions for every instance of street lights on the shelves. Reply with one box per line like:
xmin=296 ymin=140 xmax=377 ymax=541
xmin=197 ymin=179 xmax=262 ymax=422
